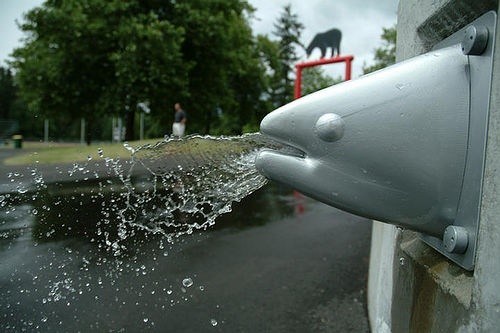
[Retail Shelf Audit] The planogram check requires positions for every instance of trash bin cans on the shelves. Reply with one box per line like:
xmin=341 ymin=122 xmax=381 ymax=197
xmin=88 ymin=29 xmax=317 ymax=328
xmin=13 ymin=135 xmax=23 ymax=149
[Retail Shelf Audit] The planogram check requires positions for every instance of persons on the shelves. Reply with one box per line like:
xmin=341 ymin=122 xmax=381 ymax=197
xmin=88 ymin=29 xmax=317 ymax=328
xmin=172 ymin=103 xmax=187 ymax=138
